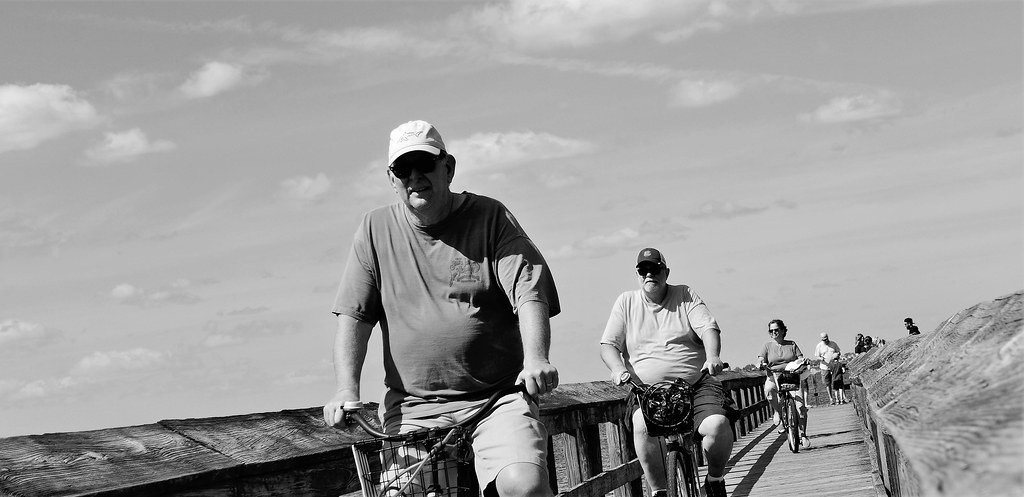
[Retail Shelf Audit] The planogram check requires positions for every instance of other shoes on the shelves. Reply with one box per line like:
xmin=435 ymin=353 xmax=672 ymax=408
xmin=773 ymin=410 xmax=781 ymax=425
xmin=801 ymin=432 xmax=810 ymax=448
xmin=830 ymin=399 xmax=835 ymax=405
xmin=836 ymin=398 xmax=850 ymax=404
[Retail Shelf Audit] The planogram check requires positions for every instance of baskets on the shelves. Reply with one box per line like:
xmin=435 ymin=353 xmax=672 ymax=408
xmin=771 ymin=371 xmax=800 ymax=391
xmin=638 ymin=386 xmax=694 ymax=437
xmin=349 ymin=426 xmax=475 ymax=497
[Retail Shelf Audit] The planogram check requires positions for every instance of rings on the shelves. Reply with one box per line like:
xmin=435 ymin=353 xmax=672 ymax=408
xmin=547 ymin=383 xmax=553 ymax=386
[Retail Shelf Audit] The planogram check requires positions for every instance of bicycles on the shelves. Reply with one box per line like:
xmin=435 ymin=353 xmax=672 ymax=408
xmin=758 ymin=357 xmax=811 ymax=456
xmin=341 ymin=379 xmax=523 ymax=497
xmin=621 ymin=360 xmax=731 ymax=497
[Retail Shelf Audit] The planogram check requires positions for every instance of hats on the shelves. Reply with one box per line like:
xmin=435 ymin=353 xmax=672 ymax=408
xmin=636 ymin=247 xmax=666 ymax=268
xmin=387 ymin=119 xmax=446 ymax=166
xmin=904 ymin=318 xmax=916 ymax=324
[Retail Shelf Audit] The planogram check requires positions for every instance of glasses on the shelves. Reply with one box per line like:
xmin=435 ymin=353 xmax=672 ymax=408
xmin=770 ymin=329 xmax=779 ymax=333
xmin=822 ymin=338 xmax=828 ymax=341
xmin=389 ymin=152 xmax=446 ymax=179
xmin=637 ymin=267 xmax=661 ymax=275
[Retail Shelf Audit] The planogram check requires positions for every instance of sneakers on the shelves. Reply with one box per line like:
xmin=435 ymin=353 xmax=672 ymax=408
xmin=704 ymin=474 xmax=727 ymax=497
xmin=653 ymin=490 xmax=668 ymax=497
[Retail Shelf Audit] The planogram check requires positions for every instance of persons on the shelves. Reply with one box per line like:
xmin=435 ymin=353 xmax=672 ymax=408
xmin=903 ymin=317 xmax=920 ymax=336
xmin=757 ymin=319 xmax=812 ymax=449
xmin=830 ymin=353 xmax=846 ymax=405
xmin=855 ymin=334 xmax=875 ymax=357
xmin=599 ymin=247 xmax=742 ymax=497
xmin=814 ymin=332 xmax=851 ymax=405
xmin=323 ymin=120 xmax=561 ymax=497
